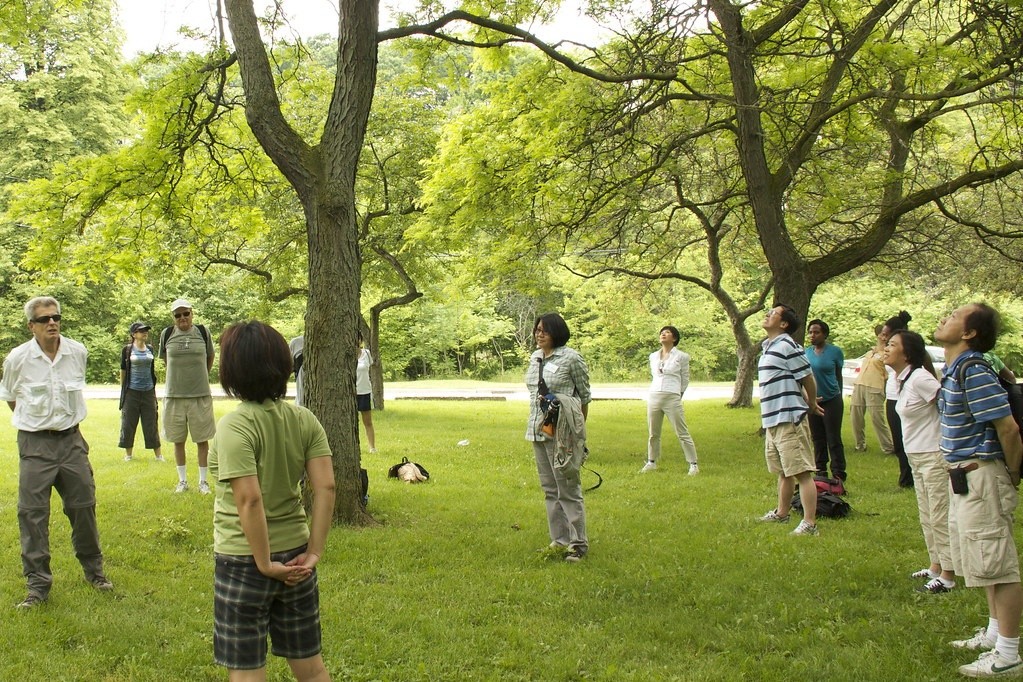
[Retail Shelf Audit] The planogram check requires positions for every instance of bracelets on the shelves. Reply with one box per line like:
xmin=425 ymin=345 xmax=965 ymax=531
xmin=1005 ymin=466 xmax=1020 ymax=473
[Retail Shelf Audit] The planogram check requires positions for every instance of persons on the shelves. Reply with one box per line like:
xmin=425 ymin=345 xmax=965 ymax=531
xmin=356 ymin=330 xmax=377 ymax=454
xmin=933 ymin=302 xmax=1023 ymax=678
xmin=756 ymin=303 xmax=825 ymax=537
xmin=213 ymin=320 xmax=336 ymax=681
xmin=119 ymin=322 xmax=164 ymax=461
xmin=525 ymin=313 xmax=592 ymax=562
xmin=802 ymin=318 xmax=848 ymax=496
xmin=639 ymin=326 xmax=700 ymax=476
xmin=850 ymin=325 xmax=894 ymax=454
xmin=881 ymin=310 xmax=938 ymax=495
xmin=883 ymin=329 xmax=957 ymax=594
xmin=158 ymin=299 xmax=215 ymax=494
xmin=288 ymin=336 xmax=305 ymax=407
xmin=0 ymin=295 xmax=114 ymax=609
xmin=982 ymin=351 xmax=1016 ymax=385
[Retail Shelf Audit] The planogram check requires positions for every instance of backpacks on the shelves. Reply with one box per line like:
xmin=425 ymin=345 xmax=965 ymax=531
xmin=387 ymin=458 xmax=430 ymax=479
xmin=791 ymin=494 xmax=849 ymax=519
xmin=793 ymin=474 xmax=847 ymax=495
xmin=961 ymin=357 xmax=1023 ymax=478
xmin=397 ymin=463 xmax=427 ymax=482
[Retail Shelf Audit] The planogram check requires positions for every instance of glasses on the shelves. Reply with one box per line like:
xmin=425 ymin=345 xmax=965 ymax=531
xmin=173 ymin=311 xmax=192 ymax=318
xmin=532 ymin=328 xmax=550 ymax=336
xmin=768 ymin=308 xmax=781 ymax=316
xmin=29 ymin=314 xmax=63 ymax=322
xmin=134 ymin=329 xmax=149 ymax=333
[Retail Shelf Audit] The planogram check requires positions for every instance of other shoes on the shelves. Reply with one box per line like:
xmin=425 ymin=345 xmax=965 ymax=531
xmin=564 ymin=550 xmax=586 ymax=563
xmin=17 ymin=593 xmax=48 ymax=607
xmin=88 ymin=575 xmax=113 ymax=590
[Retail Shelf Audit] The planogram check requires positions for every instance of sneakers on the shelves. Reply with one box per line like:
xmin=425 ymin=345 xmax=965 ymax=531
xmin=196 ymin=481 xmax=212 ymax=496
xmin=946 ymin=633 xmax=994 ymax=651
xmin=789 ymin=519 xmax=819 ymax=537
xmin=175 ymin=482 xmax=188 ymax=494
xmin=911 ymin=568 xmax=943 ymax=581
xmin=757 ymin=509 xmax=790 ymax=523
xmin=958 ymin=649 xmax=1022 ymax=677
xmin=688 ymin=463 xmax=699 ymax=477
xmin=370 ymin=448 xmax=378 ymax=452
xmin=639 ymin=461 xmax=658 ymax=475
xmin=918 ymin=578 xmax=956 ymax=594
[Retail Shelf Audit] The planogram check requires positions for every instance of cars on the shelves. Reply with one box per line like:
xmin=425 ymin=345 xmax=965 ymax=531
xmin=842 ymin=345 xmax=945 ymax=389
